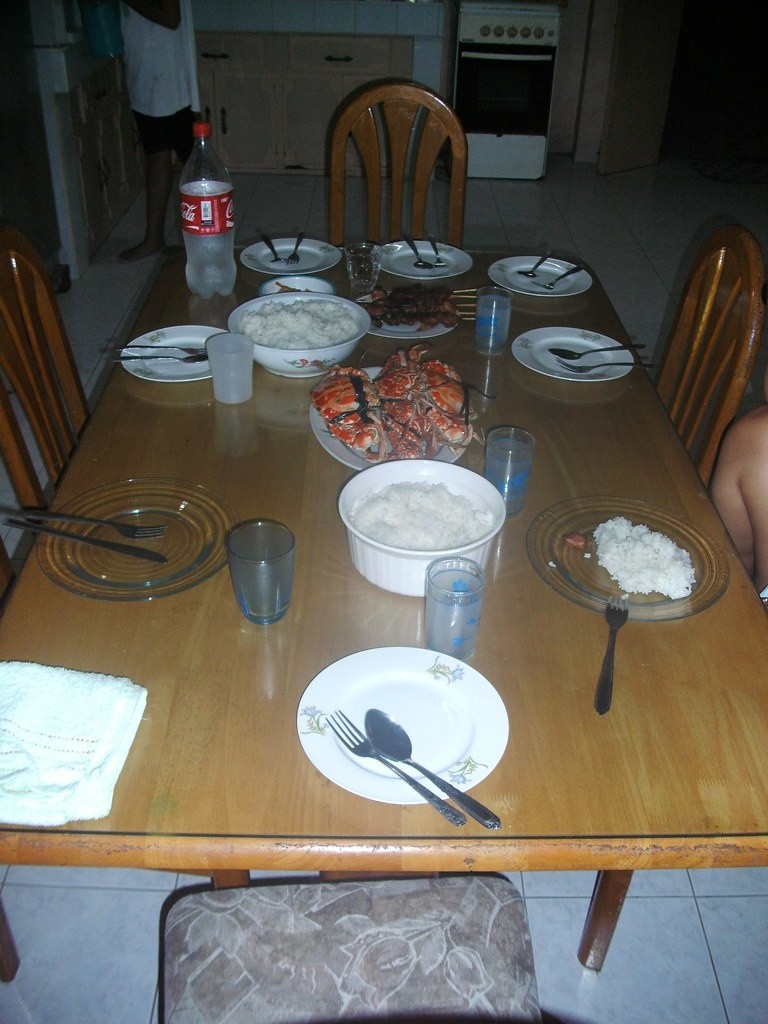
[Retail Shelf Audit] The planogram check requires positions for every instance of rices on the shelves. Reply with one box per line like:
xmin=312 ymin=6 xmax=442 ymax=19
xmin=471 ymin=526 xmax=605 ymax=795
xmin=547 ymin=516 xmax=698 ymax=600
xmin=352 ymin=480 xmax=494 ymax=550
xmin=238 ymin=299 xmax=360 ymax=349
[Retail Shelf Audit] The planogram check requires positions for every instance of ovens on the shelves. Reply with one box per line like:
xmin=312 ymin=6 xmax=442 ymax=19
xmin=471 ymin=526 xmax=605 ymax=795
xmin=450 ymin=44 xmax=555 ymax=180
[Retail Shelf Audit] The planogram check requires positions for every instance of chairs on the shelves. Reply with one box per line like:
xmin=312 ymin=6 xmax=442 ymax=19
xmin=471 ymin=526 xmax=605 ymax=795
xmin=158 ymin=875 xmax=543 ymax=1023
xmin=329 ymin=80 xmax=468 ymax=250
xmin=0 ymin=227 xmax=89 ymax=537
xmin=658 ymin=224 xmax=768 ymax=488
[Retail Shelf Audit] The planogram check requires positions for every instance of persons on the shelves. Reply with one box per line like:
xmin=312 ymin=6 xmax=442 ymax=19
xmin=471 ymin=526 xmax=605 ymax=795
xmin=707 ymin=366 xmax=768 ymax=611
xmin=116 ymin=0 xmax=201 ymax=272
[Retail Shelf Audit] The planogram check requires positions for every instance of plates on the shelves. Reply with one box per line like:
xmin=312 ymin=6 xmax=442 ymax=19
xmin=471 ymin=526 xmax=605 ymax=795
xmin=296 ymin=646 xmax=509 ymax=804
xmin=239 ymin=237 xmax=342 ymax=275
xmin=35 ymin=477 xmax=239 ymax=602
xmin=379 ymin=240 xmax=474 ymax=281
xmin=309 ymin=367 xmax=462 ymax=469
xmin=525 ymin=496 xmax=731 ymax=621
xmin=511 ymin=327 xmax=634 ymax=382
xmin=487 ymin=255 xmax=592 ymax=296
xmin=366 ymin=318 xmax=459 ymax=340
xmin=119 ymin=324 xmax=229 ymax=382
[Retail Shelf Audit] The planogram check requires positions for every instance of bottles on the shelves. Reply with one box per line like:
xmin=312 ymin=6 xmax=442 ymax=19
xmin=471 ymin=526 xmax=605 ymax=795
xmin=179 ymin=121 xmax=236 ymax=297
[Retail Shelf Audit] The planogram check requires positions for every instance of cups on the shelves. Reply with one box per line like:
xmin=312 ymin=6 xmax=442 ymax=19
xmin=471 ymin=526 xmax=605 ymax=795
xmin=226 ymin=518 xmax=295 ymax=626
xmin=206 ymin=333 xmax=254 ymax=405
xmin=424 ymin=558 xmax=487 ymax=660
xmin=344 ymin=241 xmax=382 ymax=294
xmin=484 ymin=427 xmax=536 ymax=515
xmin=473 ymin=287 xmax=512 ymax=357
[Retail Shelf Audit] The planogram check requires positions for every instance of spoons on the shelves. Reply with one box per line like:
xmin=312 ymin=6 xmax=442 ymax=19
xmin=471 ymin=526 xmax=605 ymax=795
xmin=402 ymin=234 xmax=434 ymax=270
xmin=261 ymin=233 xmax=287 ymax=263
xmin=549 ymin=344 xmax=646 ymax=360
xmin=516 ymin=250 xmax=552 ymax=277
xmin=108 ymin=355 xmax=208 ymax=363
xmin=364 ymin=708 xmax=502 ymax=829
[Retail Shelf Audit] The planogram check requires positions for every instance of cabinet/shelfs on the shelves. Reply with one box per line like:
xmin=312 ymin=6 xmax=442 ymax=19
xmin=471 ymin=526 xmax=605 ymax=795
xmin=171 ymin=29 xmax=414 ymax=179
xmin=69 ymin=52 xmax=148 ymax=264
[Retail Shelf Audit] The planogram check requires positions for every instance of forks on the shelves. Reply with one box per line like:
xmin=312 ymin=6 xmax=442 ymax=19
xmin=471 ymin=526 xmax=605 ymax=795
xmin=19 ymin=506 xmax=168 ymax=539
xmin=112 ymin=345 xmax=206 ymax=355
xmin=286 ymin=231 xmax=306 ymax=265
xmin=555 ymin=356 xmax=656 ymax=373
xmin=530 ymin=264 xmax=584 ymax=289
xmin=593 ymin=595 xmax=629 ymax=715
xmin=427 ymin=236 xmax=449 ymax=269
xmin=324 ymin=709 xmax=467 ymax=827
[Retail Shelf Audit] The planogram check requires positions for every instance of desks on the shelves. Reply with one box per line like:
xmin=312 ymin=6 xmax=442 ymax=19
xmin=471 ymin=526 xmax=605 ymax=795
xmin=0 ymin=243 xmax=768 ymax=982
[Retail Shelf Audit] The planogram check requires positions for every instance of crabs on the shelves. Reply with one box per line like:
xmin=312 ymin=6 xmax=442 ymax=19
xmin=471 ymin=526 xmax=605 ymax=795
xmin=374 ymin=342 xmax=477 ymax=462
xmin=311 ymin=362 xmax=390 ymax=464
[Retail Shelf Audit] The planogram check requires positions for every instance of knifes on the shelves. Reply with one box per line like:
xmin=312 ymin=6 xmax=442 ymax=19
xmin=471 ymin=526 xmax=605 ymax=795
xmin=3 ymin=518 xmax=169 ymax=565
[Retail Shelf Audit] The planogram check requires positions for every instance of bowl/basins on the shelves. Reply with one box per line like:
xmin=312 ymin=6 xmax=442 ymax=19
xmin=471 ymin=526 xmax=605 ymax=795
xmin=337 ymin=458 xmax=507 ymax=596
xmin=257 ymin=276 xmax=336 ymax=295
xmin=227 ymin=293 xmax=371 ymax=378
